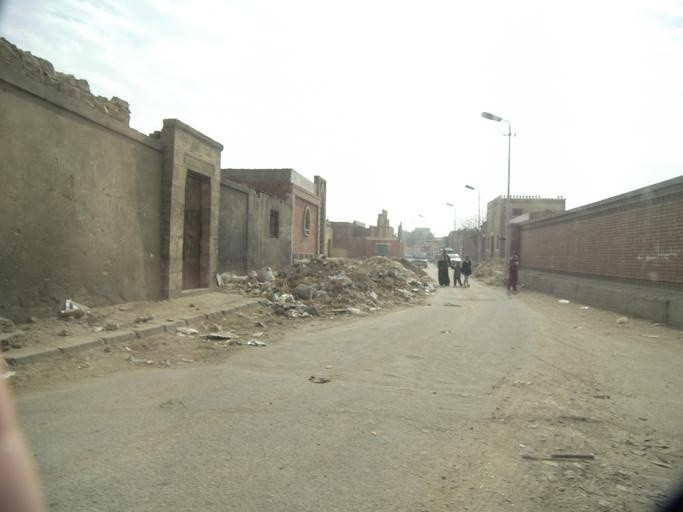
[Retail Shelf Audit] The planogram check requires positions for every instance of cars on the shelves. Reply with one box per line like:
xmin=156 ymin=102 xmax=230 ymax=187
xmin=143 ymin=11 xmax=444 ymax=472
xmin=447 ymin=254 xmax=463 ymax=269
xmin=403 ymin=258 xmax=427 ymax=268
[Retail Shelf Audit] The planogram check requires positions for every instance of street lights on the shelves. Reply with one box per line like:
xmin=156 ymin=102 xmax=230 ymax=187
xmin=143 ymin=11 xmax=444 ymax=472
xmin=482 ymin=114 xmax=511 ymax=286
xmin=447 ymin=204 xmax=456 ymax=252
xmin=466 ymin=185 xmax=481 ymax=264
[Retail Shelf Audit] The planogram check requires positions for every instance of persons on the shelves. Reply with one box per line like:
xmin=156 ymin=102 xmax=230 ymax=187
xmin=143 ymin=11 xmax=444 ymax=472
xmin=507 ymin=255 xmax=519 ymax=291
xmin=436 ymin=249 xmax=451 ymax=285
xmin=461 ymin=254 xmax=472 ymax=286
xmin=450 ymin=262 xmax=463 ymax=287
